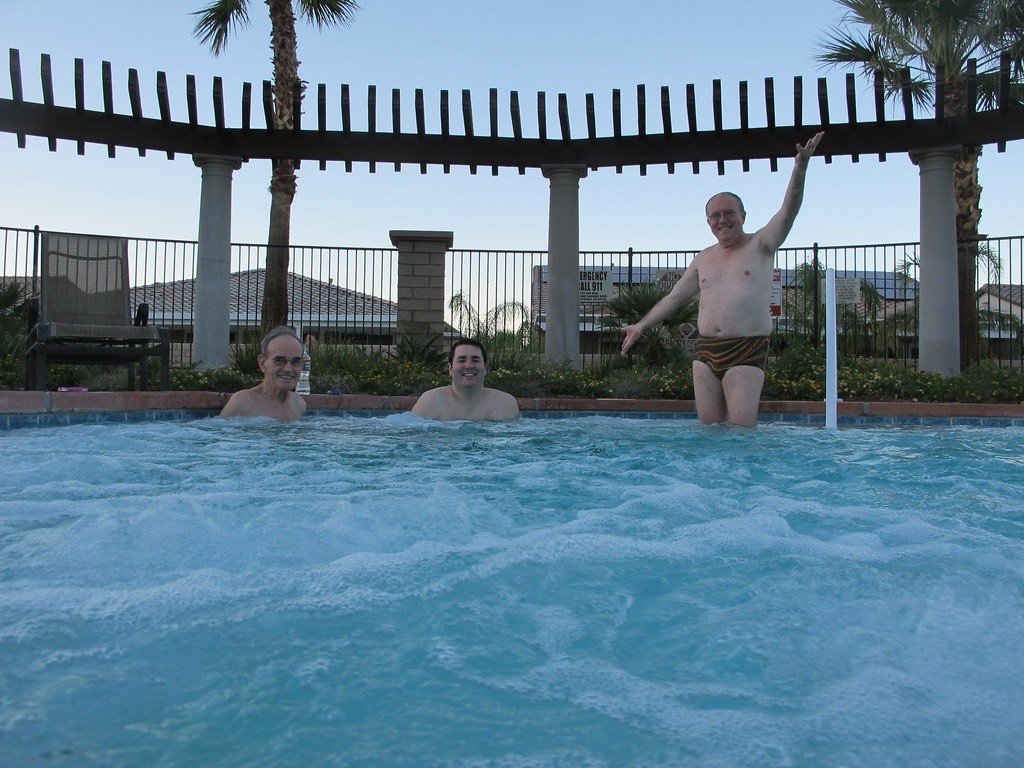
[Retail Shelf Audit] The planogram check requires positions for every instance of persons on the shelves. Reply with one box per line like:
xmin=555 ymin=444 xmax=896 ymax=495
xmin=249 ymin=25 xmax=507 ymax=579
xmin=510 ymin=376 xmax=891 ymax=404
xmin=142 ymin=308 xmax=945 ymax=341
xmin=411 ymin=337 xmax=519 ymax=422
xmin=219 ymin=325 xmax=308 ymax=424
xmin=620 ymin=131 xmax=826 ymax=427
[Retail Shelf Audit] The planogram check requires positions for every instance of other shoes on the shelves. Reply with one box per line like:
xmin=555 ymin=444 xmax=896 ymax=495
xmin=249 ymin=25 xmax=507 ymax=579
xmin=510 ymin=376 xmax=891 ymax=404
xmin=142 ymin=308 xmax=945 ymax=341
xmin=330 ymin=389 xmax=341 ymax=395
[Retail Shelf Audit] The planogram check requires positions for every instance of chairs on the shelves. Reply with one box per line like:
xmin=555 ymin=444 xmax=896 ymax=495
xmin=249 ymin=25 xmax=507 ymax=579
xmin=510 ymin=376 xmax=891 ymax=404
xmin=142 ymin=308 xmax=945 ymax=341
xmin=22 ymin=233 xmax=170 ymax=392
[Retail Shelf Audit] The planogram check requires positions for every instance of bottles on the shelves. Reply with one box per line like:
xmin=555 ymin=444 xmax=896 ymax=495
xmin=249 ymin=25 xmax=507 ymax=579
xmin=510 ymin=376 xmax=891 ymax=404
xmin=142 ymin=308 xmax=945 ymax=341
xmin=295 ymin=345 xmax=311 ymax=395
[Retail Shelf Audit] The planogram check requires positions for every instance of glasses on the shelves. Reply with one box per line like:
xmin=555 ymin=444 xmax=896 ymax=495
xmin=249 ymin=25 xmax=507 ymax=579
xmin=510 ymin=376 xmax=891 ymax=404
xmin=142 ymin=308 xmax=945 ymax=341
xmin=264 ymin=353 xmax=305 ymax=368
